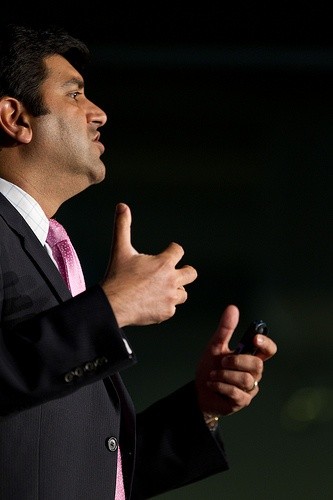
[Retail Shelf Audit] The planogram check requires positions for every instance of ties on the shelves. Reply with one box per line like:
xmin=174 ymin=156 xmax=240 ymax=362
xmin=45 ymin=219 xmax=126 ymax=500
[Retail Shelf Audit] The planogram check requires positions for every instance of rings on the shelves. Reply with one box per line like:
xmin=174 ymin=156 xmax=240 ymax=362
xmin=243 ymin=379 xmax=258 ymax=393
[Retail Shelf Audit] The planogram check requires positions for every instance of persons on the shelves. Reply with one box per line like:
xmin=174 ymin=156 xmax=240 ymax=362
xmin=0 ymin=26 xmax=277 ymax=500
